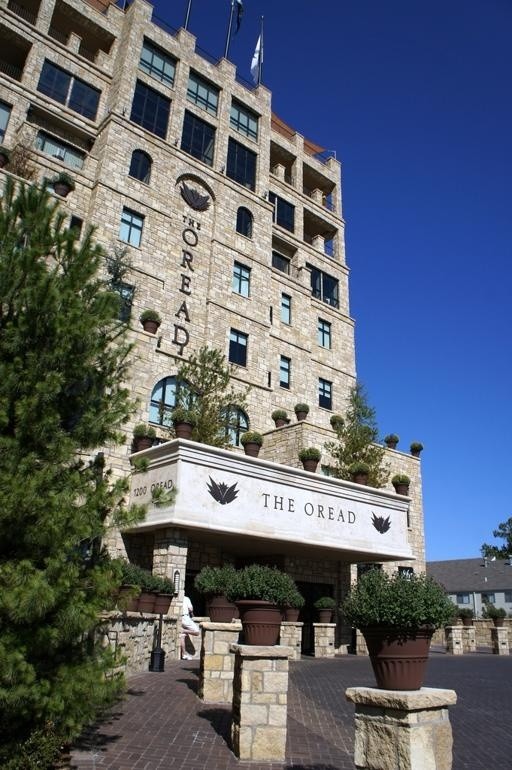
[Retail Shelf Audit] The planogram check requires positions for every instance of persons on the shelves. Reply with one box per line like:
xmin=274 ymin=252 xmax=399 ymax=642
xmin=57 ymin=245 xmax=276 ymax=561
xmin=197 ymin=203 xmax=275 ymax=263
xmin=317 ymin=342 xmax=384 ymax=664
xmin=180 ymin=589 xmax=200 ymax=661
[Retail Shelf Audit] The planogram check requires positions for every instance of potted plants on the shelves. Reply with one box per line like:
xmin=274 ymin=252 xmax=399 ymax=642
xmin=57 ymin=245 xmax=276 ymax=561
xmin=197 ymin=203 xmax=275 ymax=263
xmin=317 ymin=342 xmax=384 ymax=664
xmin=140 ymin=308 xmax=162 ymax=335
xmin=0 ymin=146 xmax=76 ymax=197
xmin=132 ymin=403 xmax=424 ymax=497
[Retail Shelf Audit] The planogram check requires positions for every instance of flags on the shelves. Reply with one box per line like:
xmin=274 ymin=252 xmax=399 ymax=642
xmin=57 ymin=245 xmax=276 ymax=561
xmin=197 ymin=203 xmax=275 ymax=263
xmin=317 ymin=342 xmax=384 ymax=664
xmin=234 ymin=1 xmax=246 ymax=33
xmin=249 ymin=33 xmax=264 ymax=86
